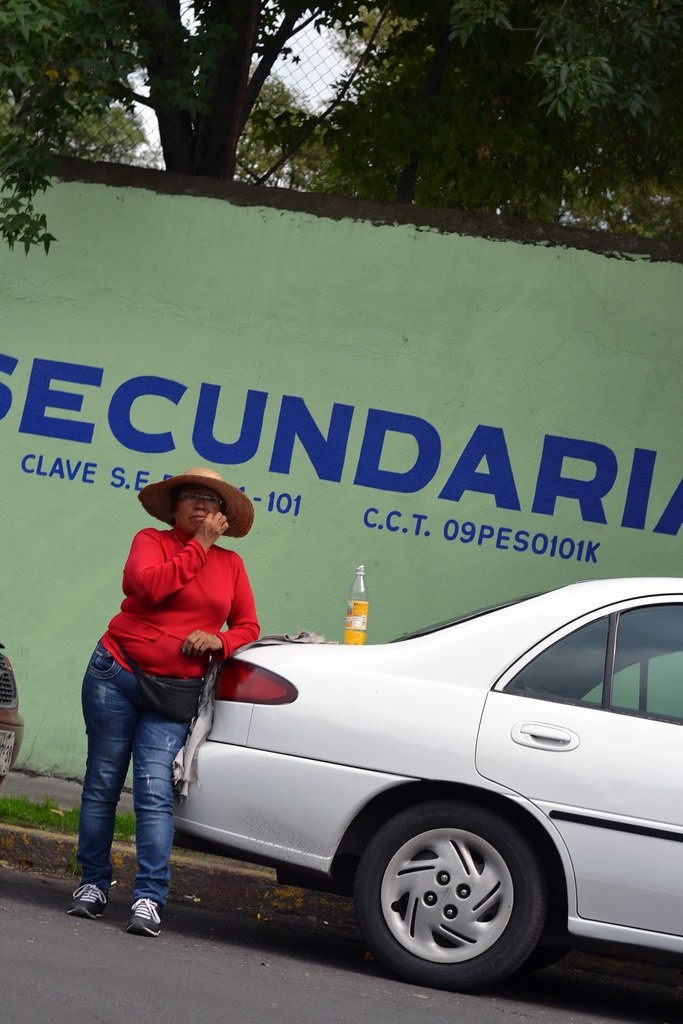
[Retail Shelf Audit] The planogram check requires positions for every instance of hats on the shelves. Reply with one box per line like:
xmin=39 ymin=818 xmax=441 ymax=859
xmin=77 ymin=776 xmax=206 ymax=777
xmin=137 ymin=467 xmax=255 ymax=538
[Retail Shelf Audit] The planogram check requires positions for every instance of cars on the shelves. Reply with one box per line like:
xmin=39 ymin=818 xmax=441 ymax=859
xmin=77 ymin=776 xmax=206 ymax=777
xmin=167 ymin=576 xmax=683 ymax=993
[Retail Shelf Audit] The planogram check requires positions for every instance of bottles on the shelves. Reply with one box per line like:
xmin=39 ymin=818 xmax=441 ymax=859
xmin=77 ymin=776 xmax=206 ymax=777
xmin=344 ymin=566 xmax=368 ymax=645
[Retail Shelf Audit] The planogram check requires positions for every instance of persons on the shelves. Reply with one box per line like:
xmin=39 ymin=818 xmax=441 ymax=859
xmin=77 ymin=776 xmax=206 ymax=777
xmin=67 ymin=468 xmax=261 ymax=936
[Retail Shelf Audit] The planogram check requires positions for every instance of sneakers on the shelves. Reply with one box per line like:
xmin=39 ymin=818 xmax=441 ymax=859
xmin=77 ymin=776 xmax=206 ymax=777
xmin=66 ymin=884 xmax=109 ymax=918
xmin=124 ymin=897 xmax=164 ymax=937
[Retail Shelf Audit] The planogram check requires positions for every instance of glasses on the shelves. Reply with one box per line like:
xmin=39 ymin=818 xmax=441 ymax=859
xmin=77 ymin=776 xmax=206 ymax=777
xmin=178 ymin=492 xmax=223 ymax=508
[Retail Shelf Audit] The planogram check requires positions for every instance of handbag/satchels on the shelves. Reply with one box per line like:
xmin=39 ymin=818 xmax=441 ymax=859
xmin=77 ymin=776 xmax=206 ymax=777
xmin=136 ymin=673 xmax=203 ymax=723
